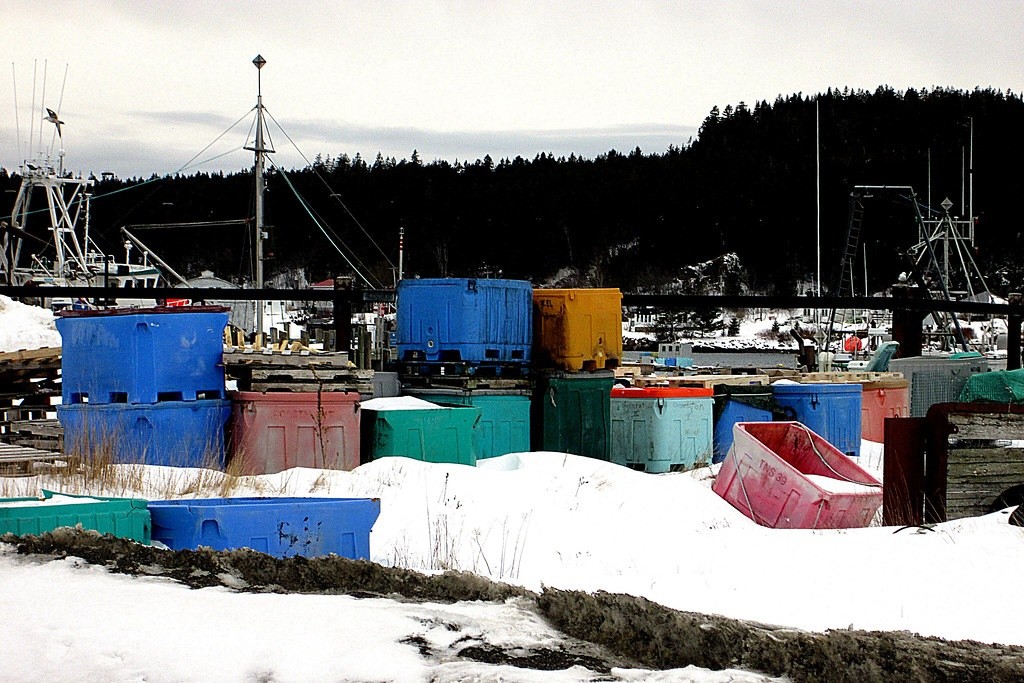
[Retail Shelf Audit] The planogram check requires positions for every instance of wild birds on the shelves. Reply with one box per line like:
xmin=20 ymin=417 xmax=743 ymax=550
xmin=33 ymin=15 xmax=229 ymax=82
xmin=42 ymin=106 xmax=65 ymax=138
xmin=897 ymin=271 xmax=907 ymax=285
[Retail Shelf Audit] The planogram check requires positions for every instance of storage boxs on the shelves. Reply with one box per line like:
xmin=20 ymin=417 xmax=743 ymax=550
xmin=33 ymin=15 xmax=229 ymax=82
xmin=0 ymin=275 xmax=913 ymax=564
